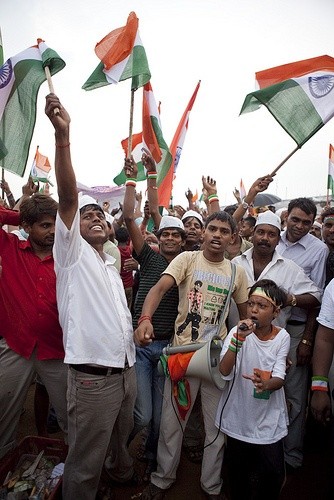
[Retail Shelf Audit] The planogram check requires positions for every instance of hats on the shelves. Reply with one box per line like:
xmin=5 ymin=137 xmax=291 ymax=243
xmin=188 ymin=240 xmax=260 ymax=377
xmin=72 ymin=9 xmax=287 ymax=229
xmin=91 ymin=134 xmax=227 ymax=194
xmin=181 ymin=210 xmax=204 ymax=227
xmin=254 ymin=210 xmax=282 ymax=232
xmin=158 ymin=215 xmax=186 ymax=231
xmin=79 ymin=195 xmax=99 ymax=210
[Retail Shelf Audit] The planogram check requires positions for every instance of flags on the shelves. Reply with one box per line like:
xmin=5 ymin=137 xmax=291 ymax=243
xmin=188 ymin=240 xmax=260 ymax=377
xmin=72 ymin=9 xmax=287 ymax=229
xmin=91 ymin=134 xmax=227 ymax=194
xmin=0 ymin=38 xmax=66 ymax=196
xmin=327 ymin=144 xmax=334 ymax=203
xmin=82 ymin=13 xmax=201 ymax=210
xmin=240 ymin=55 xmax=334 ymax=148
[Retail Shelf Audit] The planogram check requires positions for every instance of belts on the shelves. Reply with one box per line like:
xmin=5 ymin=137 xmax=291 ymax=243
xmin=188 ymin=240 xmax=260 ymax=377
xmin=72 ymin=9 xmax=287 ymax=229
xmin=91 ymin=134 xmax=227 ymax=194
xmin=70 ymin=364 xmax=122 ymax=376
xmin=286 ymin=321 xmax=305 ymax=326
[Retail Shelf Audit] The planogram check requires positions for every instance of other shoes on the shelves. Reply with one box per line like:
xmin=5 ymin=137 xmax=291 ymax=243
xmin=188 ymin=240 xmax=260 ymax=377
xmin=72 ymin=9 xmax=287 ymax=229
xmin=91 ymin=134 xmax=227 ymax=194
xmin=203 ymin=491 xmax=227 ymax=500
xmin=136 ymin=430 xmax=149 ymax=462
xmin=141 ymin=483 xmax=165 ymax=500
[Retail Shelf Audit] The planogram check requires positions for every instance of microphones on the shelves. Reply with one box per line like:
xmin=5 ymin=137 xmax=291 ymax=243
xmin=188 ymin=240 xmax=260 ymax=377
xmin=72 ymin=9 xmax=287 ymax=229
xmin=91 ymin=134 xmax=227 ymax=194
xmin=239 ymin=320 xmax=257 ymax=330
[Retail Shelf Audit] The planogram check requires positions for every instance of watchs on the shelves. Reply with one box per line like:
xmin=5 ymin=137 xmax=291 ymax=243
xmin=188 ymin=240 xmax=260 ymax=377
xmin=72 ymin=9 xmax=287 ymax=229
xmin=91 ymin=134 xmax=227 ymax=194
xmin=300 ymin=339 xmax=312 ymax=346
xmin=290 ymin=293 xmax=297 ymax=306
xmin=242 ymin=202 xmax=250 ymax=209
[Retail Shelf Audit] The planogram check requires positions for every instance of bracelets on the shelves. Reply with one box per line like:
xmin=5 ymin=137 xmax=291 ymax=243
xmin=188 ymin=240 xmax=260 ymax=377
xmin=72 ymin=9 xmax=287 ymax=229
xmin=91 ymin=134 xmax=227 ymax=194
xmin=55 ymin=143 xmax=70 ymax=148
xmin=145 ymin=186 xmax=158 ymax=191
xmin=138 ymin=315 xmax=152 ymax=326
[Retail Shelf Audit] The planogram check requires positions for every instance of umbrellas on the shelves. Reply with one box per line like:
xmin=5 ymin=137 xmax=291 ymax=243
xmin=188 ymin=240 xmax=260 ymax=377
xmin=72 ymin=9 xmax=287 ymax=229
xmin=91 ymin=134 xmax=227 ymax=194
xmin=242 ymin=195 xmax=280 ymax=207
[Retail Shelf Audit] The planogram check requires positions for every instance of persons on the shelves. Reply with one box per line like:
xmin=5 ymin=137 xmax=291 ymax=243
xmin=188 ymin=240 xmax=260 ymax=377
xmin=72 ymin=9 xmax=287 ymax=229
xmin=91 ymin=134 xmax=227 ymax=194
xmin=45 ymin=92 xmax=138 ymax=500
xmin=275 ymin=198 xmax=330 ymax=500
xmin=303 ymin=277 xmax=334 ymax=500
xmin=123 ymin=154 xmax=188 ymax=485
xmin=215 ymin=280 xmax=291 ymax=500
xmin=133 ymin=212 xmax=249 ymax=500
xmin=1 ymin=173 xmax=334 ymax=310
xmin=0 ymin=194 xmax=69 ymax=500
xmin=228 ymin=209 xmax=321 ymax=329
xmin=309 ymin=198 xmax=334 ymax=291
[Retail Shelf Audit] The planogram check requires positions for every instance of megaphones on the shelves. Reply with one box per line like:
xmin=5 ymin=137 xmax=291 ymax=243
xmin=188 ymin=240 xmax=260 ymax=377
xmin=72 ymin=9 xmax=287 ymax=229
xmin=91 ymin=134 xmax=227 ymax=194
xmin=158 ymin=335 xmax=229 ymax=391
